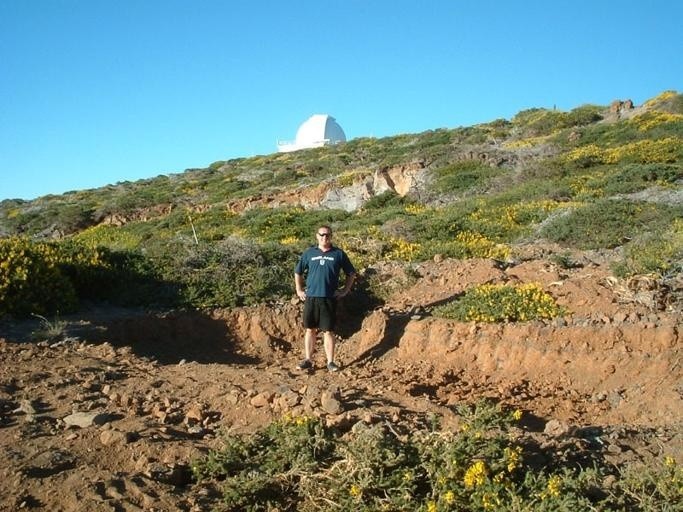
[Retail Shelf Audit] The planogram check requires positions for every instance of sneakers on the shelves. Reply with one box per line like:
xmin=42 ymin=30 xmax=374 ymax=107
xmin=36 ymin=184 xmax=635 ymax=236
xmin=297 ymin=361 xmax=311 ymax=368
xmin=327 ymin=362 xmax=338 ymax=372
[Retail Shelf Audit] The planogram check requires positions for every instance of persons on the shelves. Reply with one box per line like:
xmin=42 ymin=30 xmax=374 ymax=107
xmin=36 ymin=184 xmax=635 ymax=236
xmin=294 ymin=226 xmax=356 ymax=372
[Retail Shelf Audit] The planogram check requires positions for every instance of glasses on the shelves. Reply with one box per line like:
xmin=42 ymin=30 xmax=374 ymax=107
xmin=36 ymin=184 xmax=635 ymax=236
xmin=319 ymin=232 xmax=330 ymax=237
